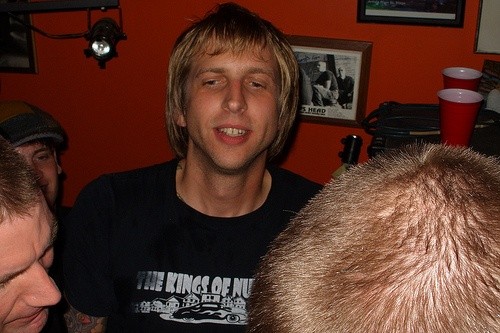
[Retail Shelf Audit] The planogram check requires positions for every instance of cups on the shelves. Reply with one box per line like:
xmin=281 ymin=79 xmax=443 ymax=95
xmin=441 ymin=66 xmax=483 ymax=90
xmin=436 ymin=88 xmax=484 ymax=146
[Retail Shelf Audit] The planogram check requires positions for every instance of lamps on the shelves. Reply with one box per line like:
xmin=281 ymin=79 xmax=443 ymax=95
xmin=83 ymin=5 xmax=126 ymax=68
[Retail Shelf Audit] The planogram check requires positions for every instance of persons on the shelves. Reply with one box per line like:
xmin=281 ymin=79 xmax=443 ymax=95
xmin=61 ymin=2 xmax=323 ymax=333
xmin=0 ymin=135 xmax=61 ymax=333
xmin=298 ymin=61 xmax=354 ymax=107
xmin=246 ymin=141 xmax=500 ymax=333
xmin=0 ymin=100 xmax=67 ymax=212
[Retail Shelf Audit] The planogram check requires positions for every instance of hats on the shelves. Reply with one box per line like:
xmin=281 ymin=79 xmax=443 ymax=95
xmin=0 ymin=100 xmax=63 ymax=148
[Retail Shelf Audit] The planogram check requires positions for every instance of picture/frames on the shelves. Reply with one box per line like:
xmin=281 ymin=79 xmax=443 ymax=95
xmin=357 ymin=0 xmax=467 ymax=28
xmin=284 ymin=34 xmax=374 ymax=127
xmin=0 ymin=9 xmax=37 ymax=75
